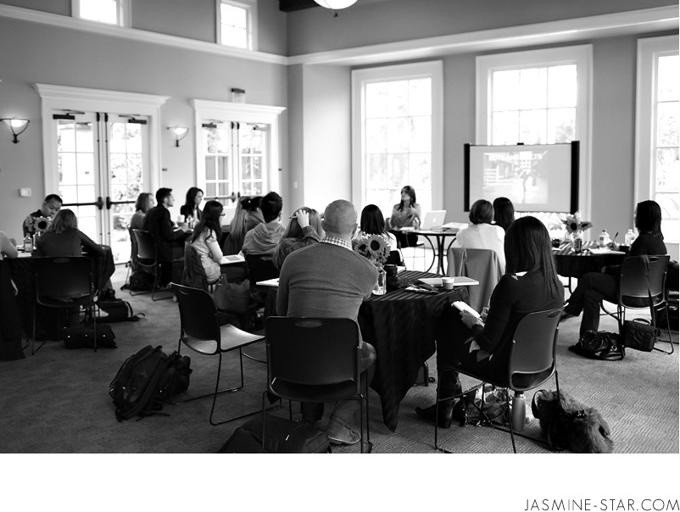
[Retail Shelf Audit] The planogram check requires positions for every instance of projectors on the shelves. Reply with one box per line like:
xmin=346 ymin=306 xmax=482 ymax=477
xmin=442 ymin=222 xmax=469 ymax=233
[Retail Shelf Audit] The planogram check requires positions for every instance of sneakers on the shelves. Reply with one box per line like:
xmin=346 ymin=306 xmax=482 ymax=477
xmin=327 ymin=426 xmax=360 ymax=444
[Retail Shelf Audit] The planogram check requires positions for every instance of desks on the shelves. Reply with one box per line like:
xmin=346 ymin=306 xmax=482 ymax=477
xmin=254 ymin=270 xmax=469 ymax=433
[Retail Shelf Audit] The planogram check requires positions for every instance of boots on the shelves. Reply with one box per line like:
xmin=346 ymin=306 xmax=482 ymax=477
xmin=414 ymin=398 xmax=453 ymax=430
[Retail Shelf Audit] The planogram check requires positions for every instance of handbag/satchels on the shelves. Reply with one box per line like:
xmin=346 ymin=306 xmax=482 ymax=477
xmin=129 ymin=272 xmax=154 ymax=291
xmin=214 ymin=278 xmax=250 ymax=314
xmin=535 ymin=388 xmax=613 ymax=453
xmin=622 ymin=320 xmax=657 ymax=353
xmin=466 ymin=390 xmax=509 ymax=425
xmin=220 ymin=414 xmax=330 ymax=453
xmin=94 ymin=291 xmax=135 ymax=322
xmin=66 ymin=323 xmax=117 ymax=351
xmin=579 ymin=329 xmax=625 ymax=362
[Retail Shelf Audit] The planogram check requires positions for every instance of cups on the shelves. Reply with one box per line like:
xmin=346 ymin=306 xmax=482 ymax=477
xmin=383 ymin=264 xmax=397 ymax=288
xmin=178 ymin=215 xmax=185 ymax=223
xmin=574 ymin=239 xmax=582 ymax=253
xmin=442 ymin=277 xmax=454 ymax=290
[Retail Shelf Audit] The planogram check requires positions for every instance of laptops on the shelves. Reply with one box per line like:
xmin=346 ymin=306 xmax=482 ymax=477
xmin=420 ymin=210 xmax=447 ymax=230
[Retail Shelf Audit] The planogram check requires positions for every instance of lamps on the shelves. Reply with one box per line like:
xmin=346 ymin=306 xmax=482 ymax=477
xmin=1 ymin=116 xmax=31 ymax=145
xmin=166 ymin=125 xmax=189 ymax=146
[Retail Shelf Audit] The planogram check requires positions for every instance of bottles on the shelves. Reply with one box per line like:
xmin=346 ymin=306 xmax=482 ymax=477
xmin=187 ymin=215 xmax=193 ymax=229
xmin=413 ymin=217 xmax=420 ymax=231
xmin=624 ymin=229 xmax=634 ymax=248
xmin=599 ymin=229 xmax=611 ymax=247
xmin=371 ymin=263 xmax=387 ymax=296
xmin=23 ymin=233 xmax=33 ymax=253
xmin=32 ymin=232 xmax=39 ymax=250
xmin=511 ymin=391 xmax=527 ymax=432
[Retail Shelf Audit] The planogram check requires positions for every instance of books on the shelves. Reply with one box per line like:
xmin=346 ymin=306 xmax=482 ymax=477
xmin=419 ymin=275 xmax=480 ymax=288
xmin=588 ymin=247 xmax=627 ymax=255
xmin=2 ymin=252 xmax=32 ymax=258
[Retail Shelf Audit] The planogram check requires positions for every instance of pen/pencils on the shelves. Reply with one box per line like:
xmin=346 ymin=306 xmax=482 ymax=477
xmin=289 ymin=216 xmax=297 ymax=218
xmin=612 ymin=233 xmax=618 ymax=242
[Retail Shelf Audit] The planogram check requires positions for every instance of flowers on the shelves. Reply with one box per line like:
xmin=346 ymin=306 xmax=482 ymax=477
xmin=561 ymin=211 xmax=593 ymax=236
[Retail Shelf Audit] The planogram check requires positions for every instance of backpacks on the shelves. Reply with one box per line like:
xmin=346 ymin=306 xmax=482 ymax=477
xmin=109 ymin=345 xmax=191 ymax=422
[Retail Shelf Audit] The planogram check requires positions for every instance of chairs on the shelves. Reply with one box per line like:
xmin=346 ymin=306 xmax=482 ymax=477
xmin=1 ymin=243 xmax=118 ymax=356
xmin=169 ymin=281 xmax=284 ymax=426
xmin=431 ymin=304 xmax=570 ymax=453
xmin=124 ymin=220 xmax=679 ymax=359
xmin=258 ymin=313 xmax=376 ymax=454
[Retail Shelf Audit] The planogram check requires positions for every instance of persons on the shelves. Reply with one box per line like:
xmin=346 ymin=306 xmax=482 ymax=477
xmin=564 ymin=201 xmax=667 ymax=344
xmin=0 ymin=232 xmax=20 ymax=259
xmin=493 ymin=196 xmax=515 ymax=231
xmin=23 ymin=194 xmax=63 ymax=237
xmin=126 ymin=187 xmax=382 ymax=446
xmin=356 ymin=203 xmax=398 ymax=251
xmin=387 ymin=185 xmax=421 ymax=248
xmin=36 ymin=207 xmax=98 ymax=332
xmin=414 ymin=215 xmax=564 ymax=429
xmin=456 ymin=199 xmax=505 ymax=274
